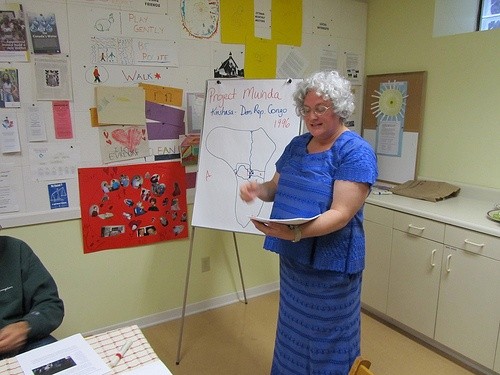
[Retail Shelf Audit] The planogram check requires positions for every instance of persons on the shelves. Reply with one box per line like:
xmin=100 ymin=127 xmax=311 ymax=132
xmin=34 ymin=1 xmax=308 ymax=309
xmin=48 ymin=74 xmax=58 ymax=87
xmin=0 ymin=11 xmax=56 ymax=50
xmin=90 ymin=171 xmax=186 ymax=239
xmin=1 ymin=73 xmax=17 ymax=105
xmin=237 ymin=71 xmax=378 ymax=374
xmin=0 ymin=235 xmax=63 ymax=361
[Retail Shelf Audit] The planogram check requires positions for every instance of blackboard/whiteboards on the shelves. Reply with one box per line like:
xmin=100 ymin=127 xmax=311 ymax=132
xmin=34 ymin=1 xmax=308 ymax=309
xmin=190 ymin=78 xmax=309 ymax=237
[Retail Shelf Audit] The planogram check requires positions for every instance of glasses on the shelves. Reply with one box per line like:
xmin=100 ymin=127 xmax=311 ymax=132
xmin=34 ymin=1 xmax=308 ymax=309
xmin=299 ymin=105 xmax=332 ymax=116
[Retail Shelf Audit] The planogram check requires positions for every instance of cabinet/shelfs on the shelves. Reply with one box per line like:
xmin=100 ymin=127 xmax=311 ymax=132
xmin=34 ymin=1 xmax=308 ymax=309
xmin=360 ymin=202 xmax=500 ymax=375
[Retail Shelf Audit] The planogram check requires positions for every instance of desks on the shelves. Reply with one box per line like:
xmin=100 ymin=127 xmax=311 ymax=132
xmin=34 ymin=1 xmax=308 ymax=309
xmin=0 ymin=325 xmax=173 ymax=375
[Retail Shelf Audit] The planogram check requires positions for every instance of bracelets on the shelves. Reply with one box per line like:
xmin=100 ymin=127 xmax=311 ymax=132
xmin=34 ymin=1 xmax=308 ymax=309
xmin=290 ymin=224 xmax=301 ymax=244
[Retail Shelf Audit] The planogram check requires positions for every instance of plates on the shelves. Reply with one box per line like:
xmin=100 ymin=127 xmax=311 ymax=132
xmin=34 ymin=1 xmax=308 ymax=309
xmin=487 ymin=209 xmax=500 ymax=222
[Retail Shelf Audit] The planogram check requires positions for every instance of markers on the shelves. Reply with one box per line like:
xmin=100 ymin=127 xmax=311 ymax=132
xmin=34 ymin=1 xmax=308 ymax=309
xmin=375 ymin=191 xmax=393 ymax=196
xmin=109 ymin=337 xmax=134 ymax=368
